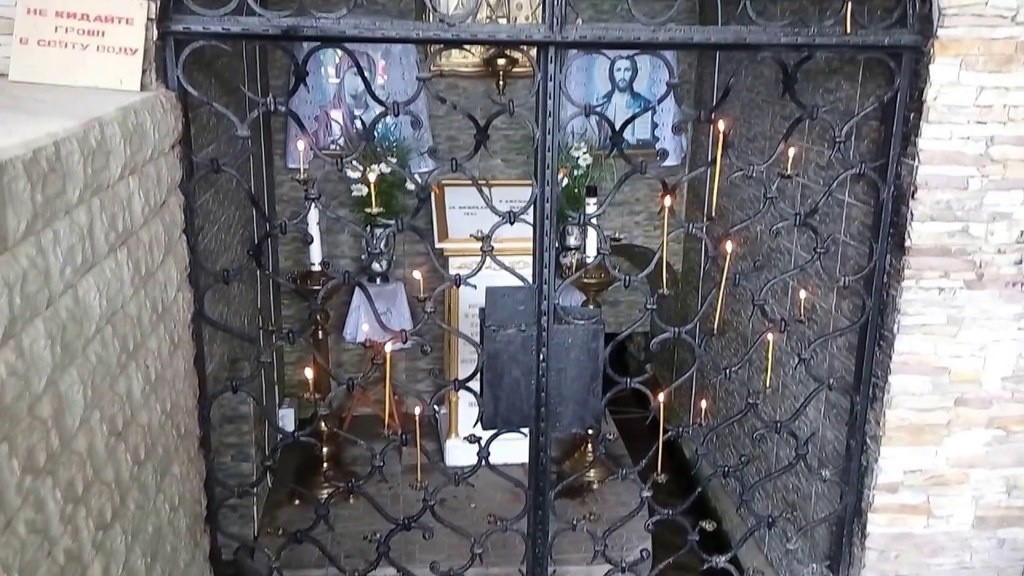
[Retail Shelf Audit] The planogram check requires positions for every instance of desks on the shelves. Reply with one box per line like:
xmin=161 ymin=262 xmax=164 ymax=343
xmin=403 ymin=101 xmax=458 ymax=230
xmin=350 ymin=282 xmax=411 ymax=440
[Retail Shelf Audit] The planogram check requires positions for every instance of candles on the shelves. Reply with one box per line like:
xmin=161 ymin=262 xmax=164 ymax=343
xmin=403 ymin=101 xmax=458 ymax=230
xmin=294 ymin=140 xmax=427 ymax=486
xmin=657 ymin=122 xmax=807 ymax=486
xmin=349 ymin=138 xmax=406 ymax=282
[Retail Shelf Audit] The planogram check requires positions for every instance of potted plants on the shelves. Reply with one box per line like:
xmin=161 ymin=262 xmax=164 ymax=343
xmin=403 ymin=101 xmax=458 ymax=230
xmin=548 ymin=139 xmax=585 ymax=275
